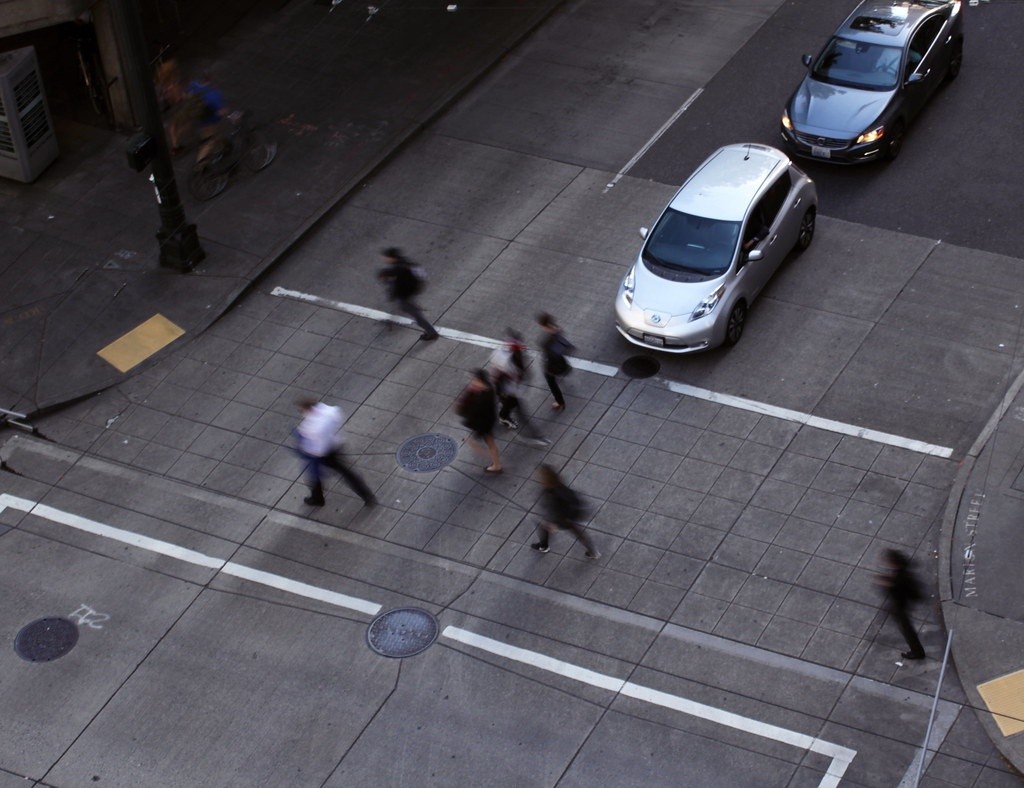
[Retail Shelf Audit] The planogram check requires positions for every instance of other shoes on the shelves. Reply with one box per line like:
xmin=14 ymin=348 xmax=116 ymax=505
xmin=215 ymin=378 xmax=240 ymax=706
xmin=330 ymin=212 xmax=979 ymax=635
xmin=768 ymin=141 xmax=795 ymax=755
xmin=901 ymin=650 xmax=925 ymax=659
xmin=585 ymin=551 xmax=601 ymax=558
xmin=532 ymin=543 xmax=550 ymax=552
xmin=484 ymin=465 xmax=504 ymax=472
xmin=499 ymin=418 xmax=518 ymax=429
xmin=305 ymin=497 xmax=325 ymax=506
xmin=420 ymin=333 xmax=438 ymax=340
xmin=552 ymin=402 xmax=565 ymax=410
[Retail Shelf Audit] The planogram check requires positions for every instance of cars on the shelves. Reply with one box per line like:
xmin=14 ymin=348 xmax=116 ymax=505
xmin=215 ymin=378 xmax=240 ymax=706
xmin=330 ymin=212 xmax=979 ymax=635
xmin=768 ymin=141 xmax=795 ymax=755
xmin=779 ymin=1 xmax=967 ymax=167
xmin=612 ymin=142 xmax=820 ymax=353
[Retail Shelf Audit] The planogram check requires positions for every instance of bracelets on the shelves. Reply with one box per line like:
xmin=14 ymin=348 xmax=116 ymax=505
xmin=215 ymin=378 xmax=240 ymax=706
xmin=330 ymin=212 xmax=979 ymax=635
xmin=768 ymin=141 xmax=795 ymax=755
xmin=752 ymin=238 xmax=756 ymax=242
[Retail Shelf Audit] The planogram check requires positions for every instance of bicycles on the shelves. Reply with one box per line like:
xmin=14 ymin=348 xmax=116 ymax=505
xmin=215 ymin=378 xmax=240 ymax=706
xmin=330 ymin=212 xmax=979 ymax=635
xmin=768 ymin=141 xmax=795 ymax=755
xmin=185 ymin=115 xmax=277 ymax=201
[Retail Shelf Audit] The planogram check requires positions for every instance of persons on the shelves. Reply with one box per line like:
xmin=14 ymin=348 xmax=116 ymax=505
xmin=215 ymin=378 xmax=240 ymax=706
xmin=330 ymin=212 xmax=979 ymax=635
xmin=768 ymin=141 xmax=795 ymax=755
xmin=875 ymin=47 xmax=921 ymax=75
xmin=530 ymin=464 xmax=602 ymax=560
xmin=742 ymin=213 xmax=770 ymax=250
xmin=377 ymin=249 xmax=439 ymax=340
xmin=879 ymin=549 xmax=925 ymax=659
xmin=454 ymin=313 xmax=574 ymax=473
xmin=158 ymin=48 xmax=222 ymax=125
xmin=291 ymin=394 xmax=378 ymax=508
